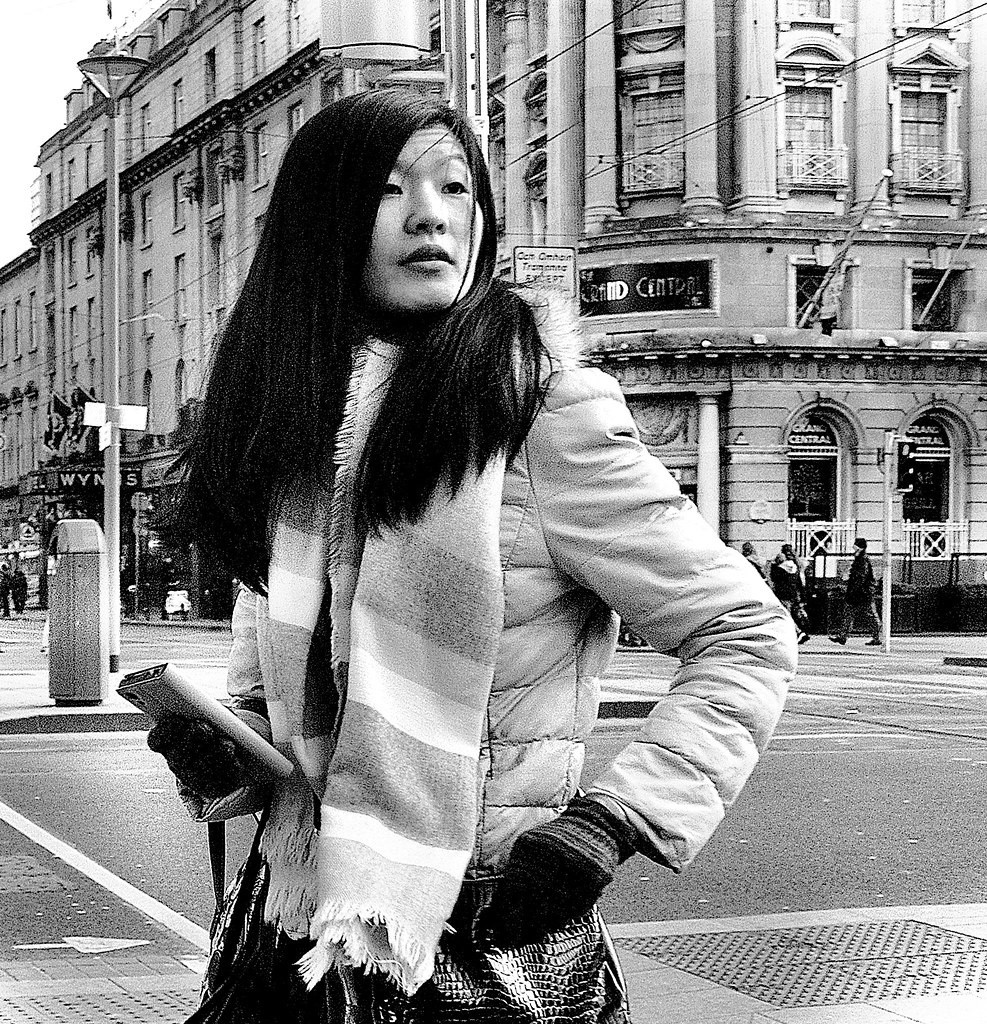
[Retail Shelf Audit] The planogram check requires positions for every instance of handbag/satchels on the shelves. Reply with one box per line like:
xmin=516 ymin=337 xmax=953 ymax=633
xmin=297 ymin=856 xmax=633 ymax=1024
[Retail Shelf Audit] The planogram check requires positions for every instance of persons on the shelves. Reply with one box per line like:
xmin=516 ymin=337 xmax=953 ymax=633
xmin=0 ymin=564 xmax=29 ymax=617
xmin=150 ymin=91 xmax=798 ymax=1024
xmin=742 ymin=537 xmax=883 ymax=644
xmin=156 ymin=555 xmax=242 ymax=622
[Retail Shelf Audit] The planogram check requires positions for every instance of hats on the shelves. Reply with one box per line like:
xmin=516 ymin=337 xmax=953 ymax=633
xmin=855 ymin=538 xmax=867 ymax=549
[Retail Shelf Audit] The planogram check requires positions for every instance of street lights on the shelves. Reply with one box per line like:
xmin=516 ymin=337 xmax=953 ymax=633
xmin=77 ymin=54 xmax=154 ymax=673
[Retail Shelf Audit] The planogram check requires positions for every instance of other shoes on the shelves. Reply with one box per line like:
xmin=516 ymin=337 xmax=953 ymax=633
xmin=865 ymin=636 xmax=881 ymax=645
xmin=797 ymin=633 xmax=809 ymax=644
xmin=828 ymin=637 xmax=846 ymax=645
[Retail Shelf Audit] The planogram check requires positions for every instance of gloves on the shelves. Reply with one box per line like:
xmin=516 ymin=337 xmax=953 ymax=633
xmin=147 ymin=713 xmax=249 ymax=794
xmin=466 ymin=797 xmax=642 ymax=966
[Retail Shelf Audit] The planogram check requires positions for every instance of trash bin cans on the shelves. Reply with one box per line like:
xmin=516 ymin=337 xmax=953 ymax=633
xmin=45 ymin=518 xmax=109 ymax=706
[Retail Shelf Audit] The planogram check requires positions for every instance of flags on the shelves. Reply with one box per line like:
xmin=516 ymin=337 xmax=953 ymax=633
xmin=44 ymin=388 xmax=102 ymax=457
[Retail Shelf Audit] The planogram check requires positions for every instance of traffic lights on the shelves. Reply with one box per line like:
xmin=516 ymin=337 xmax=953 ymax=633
xmin=897 ymin=439 xmax=918 ymax=489
